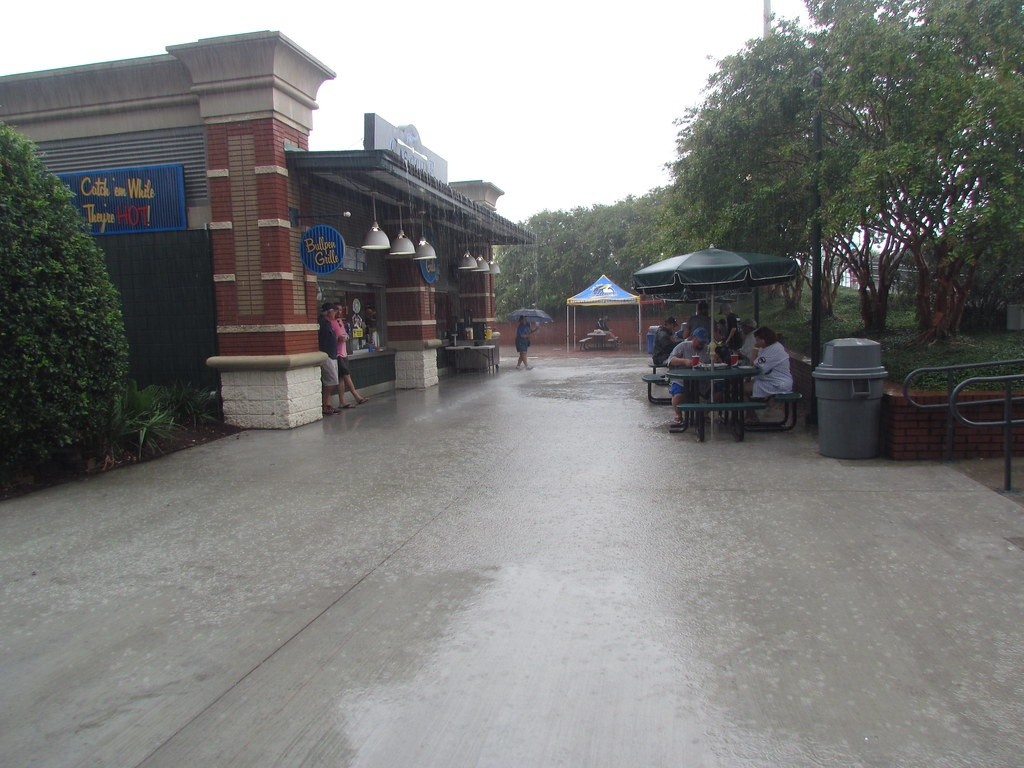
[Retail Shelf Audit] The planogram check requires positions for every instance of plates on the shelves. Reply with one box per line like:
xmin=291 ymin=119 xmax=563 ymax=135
xmin=738 ymin=365 xmax=753 ymax=369
xmin=701 ymin=362 xmax=728 ymax=371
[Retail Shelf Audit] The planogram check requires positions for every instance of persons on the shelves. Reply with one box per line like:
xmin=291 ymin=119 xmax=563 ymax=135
xmin=515 ymin=316 xmax=539 ymax=370
xmin=597 ymin=314 xmax=623 ymax=344
xmin=317 ymin=302 xmax=369 ymax=415
xmin=652 ymin=302 xmax=793 ymax=425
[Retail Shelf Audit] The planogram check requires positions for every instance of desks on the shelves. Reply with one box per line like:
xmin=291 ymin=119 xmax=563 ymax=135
xmin=669 ymin=367 xmax=759 ymax=432
xmin=587 ymin=330 xmax=609 ymax=351
xmin=445 ymin=344 xmax=495 ymax=376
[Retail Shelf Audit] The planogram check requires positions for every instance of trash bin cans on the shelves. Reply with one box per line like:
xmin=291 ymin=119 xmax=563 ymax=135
xmin=647 ymin=332 xmax=655 ymax=354
xmin=812 ymin=337 xmax=888 ymax=459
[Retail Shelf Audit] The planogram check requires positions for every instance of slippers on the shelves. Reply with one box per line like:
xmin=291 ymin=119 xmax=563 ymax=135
xmin=359 ymin=397 xmax=368 ymax=404
xmin=338 ymin=404 xmax=356 ymax=408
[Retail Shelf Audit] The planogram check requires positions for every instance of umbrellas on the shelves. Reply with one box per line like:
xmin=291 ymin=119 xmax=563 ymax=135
xmin=633 ymin=244 xmax=799 ymax=430
xmin=507 ymin=309 xmax=553 ymax=329
xmin=651 ymin=287 xmax=752 ymax=303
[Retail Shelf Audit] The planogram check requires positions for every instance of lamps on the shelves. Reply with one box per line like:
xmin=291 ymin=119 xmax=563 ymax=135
xmin=361 ymin=193 xmax=391 ymax=250
xmin=485 ymin=242 xmax=501 ymax=274
xmin=390 ymin=204 xmax=416 ymax=254
xmin=413 ymin=212 xmax=437 ymax=260
xmin=458 ymin=231 xmax=478 ymax=269
xmin=470 ymin=235 xmax=490 ymax=271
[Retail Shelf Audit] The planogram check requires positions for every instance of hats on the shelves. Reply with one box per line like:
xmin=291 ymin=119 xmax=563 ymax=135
xmin=693 ymin=327 xmax=710 ymax=342
xmin=667 ymin=316 xmax=679 ymax=326
xmin=518 ymin=315 xmax=527 ymax=322
xmin=322 ymin=303 xmax=337 ymax=312
xmin=718 ymin=303 xmax=731 ymax=315
xmin=739 ymin=318 xmax=757 ymax=329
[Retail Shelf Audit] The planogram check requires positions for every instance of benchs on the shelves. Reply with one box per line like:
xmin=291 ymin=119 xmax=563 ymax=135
xmin=748 ymin=392 xmax=802 ymax=432
xmin=677 ymin=403 xmax=767 ymax=442
xmin=579 ymin=337 xmax=592 ymax=351
xmin=608 ymin=337 xmax=618 ymax=350
xmin=648 ymin=364 xmax=668 ymax=375
xmin=641 ymin=373 xmax=672 ymax=405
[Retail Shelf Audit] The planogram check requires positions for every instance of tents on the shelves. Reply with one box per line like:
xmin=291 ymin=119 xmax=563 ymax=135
xmin=566 ymin=274 xmax=641 ymax=352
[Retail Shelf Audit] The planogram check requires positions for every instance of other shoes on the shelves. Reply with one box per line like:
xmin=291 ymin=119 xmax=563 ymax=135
xmin=744 ymin=416 xmax=760 ymax=423
xmin=705 ymin=416 xmax=711 ymax=423
xmin=322 ymin=405 xmax=341 ymax=416
xmin=674 ymin=416 xmax=683 ymax=425
xmin=516 ymin=366 xmax=524 ymax=370
xmin=525 ymin=366 xmax=533 ymax=370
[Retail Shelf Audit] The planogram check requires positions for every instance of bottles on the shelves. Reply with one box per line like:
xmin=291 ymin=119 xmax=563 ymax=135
xmin=486 ymin=327 xmax=492 ymax=339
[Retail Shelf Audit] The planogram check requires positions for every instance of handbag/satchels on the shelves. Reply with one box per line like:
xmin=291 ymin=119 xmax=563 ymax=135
xmin=527 ymin=339 xmax=530 ymax=347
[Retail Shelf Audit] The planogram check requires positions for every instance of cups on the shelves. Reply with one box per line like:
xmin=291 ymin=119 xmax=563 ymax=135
xmin=730 ymin=355 xmax=739 ymax=367
xmin=692 ymin=356 xmax=700 ymax=366
